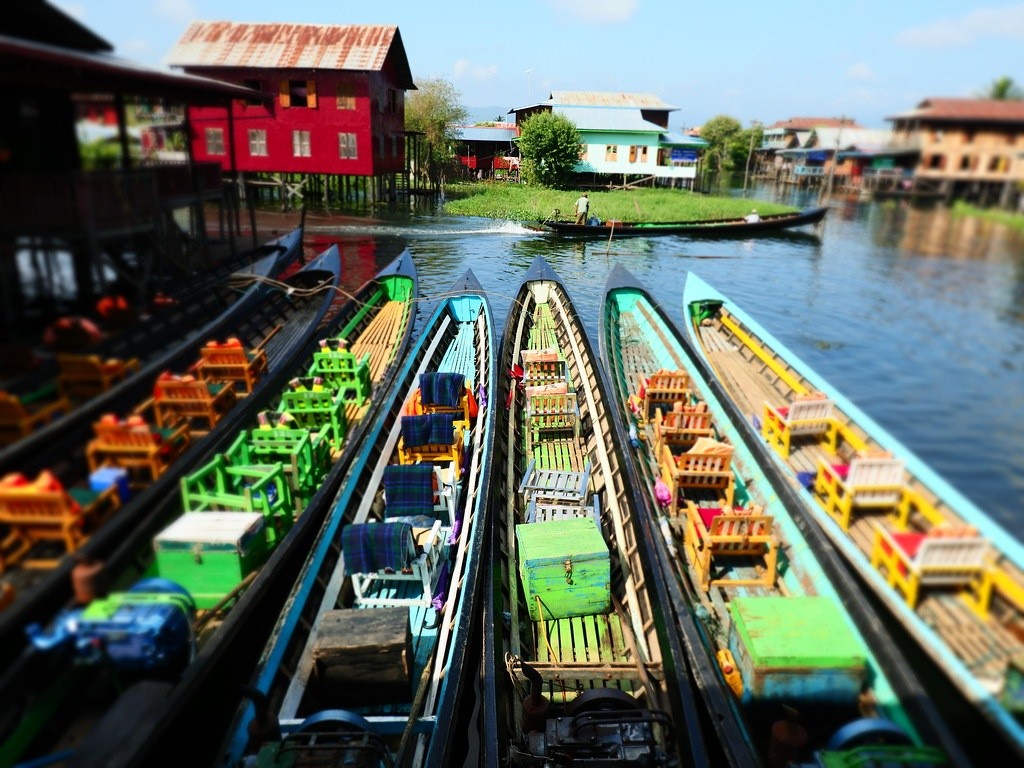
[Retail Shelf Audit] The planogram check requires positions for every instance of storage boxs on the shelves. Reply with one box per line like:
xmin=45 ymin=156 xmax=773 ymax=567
xmin=606 ymin=219 xmax=623 ymax=227
xmin=728 ymin=595 xmax=869 ymax=725
xmin=87 ymin=468 xmax=128 ymax=505
xmin=311 ymin=606 xmax=413 ymax=708
xmin=515 ymin=517 xmax=611 ymax=623
xmin=152 ymin=511 xmax=268 ymax=612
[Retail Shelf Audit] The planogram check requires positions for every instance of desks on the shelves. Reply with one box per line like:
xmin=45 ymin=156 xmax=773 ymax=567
xmin=179 ymin=428 xmax=318 ymax=553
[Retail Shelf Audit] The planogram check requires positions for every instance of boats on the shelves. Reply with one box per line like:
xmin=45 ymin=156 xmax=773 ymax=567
xmin=483 ymin=253 xmax=761 ymax=768
xmin=215 ymin=267 xmax=496 ymax=768
xmin=680 ymin=271 xmax=1024 ymax=749
xmin=0 ymin=247 xmax=418 ymax=768
xmin=596 ymin=262 xmax=953 ymax=768
xmin=520 ymin=206 xmax=829 ymax=239
xmin=0 ymin=226 xmax=345 ymax=665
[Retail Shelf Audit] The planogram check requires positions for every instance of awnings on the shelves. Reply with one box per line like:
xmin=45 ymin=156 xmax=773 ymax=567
xmin=776 ymin=146 xmax=920 ymax=164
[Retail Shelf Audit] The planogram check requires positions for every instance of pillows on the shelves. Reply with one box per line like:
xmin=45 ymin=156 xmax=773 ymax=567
xmin=525 ymin=382 xmax=567 ymax=423
xmin=794 ymin=391 xmax=827 ymax=402
xmin=519 ymin=348 xmax=559 ymax=384
xmin=928 ymin=522 xmax=979 ymax=537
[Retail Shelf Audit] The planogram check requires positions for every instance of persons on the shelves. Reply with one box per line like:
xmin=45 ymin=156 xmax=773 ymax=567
xmin=742 ymin=208 xmax=761 ymax=224
xmin=573 ymin=193 xmax=590 ymax=226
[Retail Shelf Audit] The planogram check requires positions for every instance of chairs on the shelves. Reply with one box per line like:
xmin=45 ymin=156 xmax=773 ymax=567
xmin=526 ymin=495 xmax=602 ymax=533
xmin=340 ymin=516 xmax=448 ymax=608
xmin=85 ymin=412 xmax=192 ymax=492
xmin=760 ymin=390 xmax=840 ymax=459
xmin=252 ymin=423 xmax=336 ymax=496
xmin=280 ymin=386 xmax=351 ymax=452
xmin=814 ymin=453 xmax=914 ymax=534
xmin=406 ymin=372 xmax=478 ymax=433
xmin=519 ymin=459 xmax=592 ymax=506
xmin=660 ymin=444 xmax=738 ymax=516
xmin=193 ymin=338 xmax=268 ymax=396
xmin=872 ymin=523 xmax=1005 ymax=621
xmin=653 ymin=406 xmax=714 ymax=456
xmin=382 ymin=461 xmax=463 ymax=526
xmin=0 ymin=390 xmax=73 ymax=445
xmin=640 ymin=374 xmax=693 ymax=423
xmin=152 ymin=370 xmax=236 ymax=436
xmin=685 ymin=499 xmax=780 ymax=592
xmin=398 ymin=413 xmax=466 ymax=482
xmin=525 ymin=359 xmax=569 ymax=389
xmin=527 ymin=392 xmax=580 ymax=452
xmin=311 ymin=352 xmax=373 ymax=407
xmin=51 ymin=349 xmax=137 ymax=405
xmin=0 ymin=468 xmax=123 ymax=575
